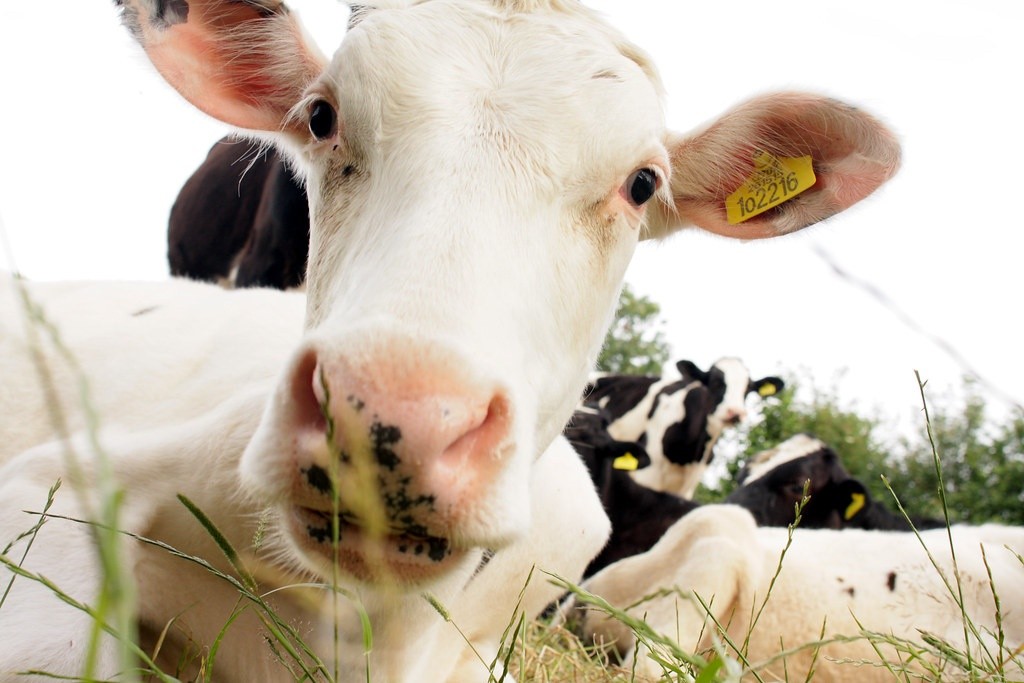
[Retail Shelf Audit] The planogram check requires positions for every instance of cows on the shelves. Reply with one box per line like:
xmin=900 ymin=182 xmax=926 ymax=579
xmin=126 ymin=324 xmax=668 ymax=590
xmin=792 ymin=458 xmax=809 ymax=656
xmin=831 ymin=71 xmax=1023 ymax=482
xmin=0 ymin=0 xmax=1024 ymax=683
